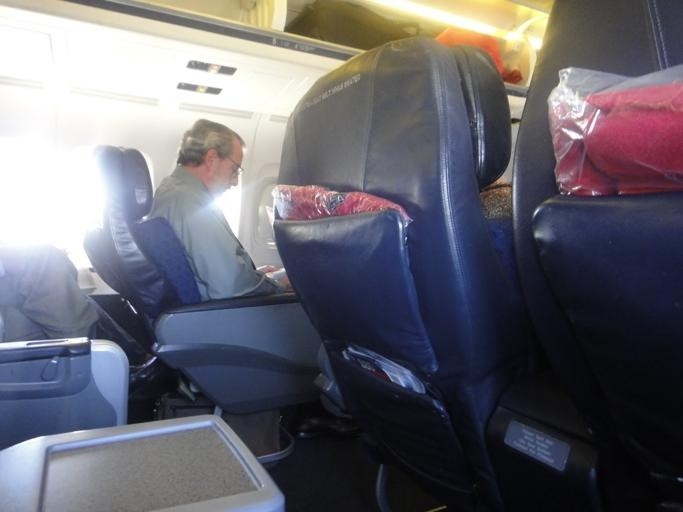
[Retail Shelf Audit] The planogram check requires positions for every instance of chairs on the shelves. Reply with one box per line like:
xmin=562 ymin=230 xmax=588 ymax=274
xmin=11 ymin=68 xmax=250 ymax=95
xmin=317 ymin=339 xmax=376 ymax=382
xmin=277 ymin=35 xmax=520 ymax=507
xmin=509 ymin=2 xmax=679 ymax=507
xmin=83 ymin=142 xmax=316 ymax=440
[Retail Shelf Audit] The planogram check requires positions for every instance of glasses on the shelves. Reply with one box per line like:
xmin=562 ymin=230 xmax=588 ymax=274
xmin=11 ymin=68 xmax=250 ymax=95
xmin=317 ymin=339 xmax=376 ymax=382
xmin=225 ymin=154 xmax=248 ymax=175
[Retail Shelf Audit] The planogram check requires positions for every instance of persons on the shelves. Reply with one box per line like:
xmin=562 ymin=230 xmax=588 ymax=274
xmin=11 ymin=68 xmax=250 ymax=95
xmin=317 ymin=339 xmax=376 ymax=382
xmin=148 ymin=117 xmax=295 ymax=302
xmin=0 ymin=242 xmax=153 ymax=366
